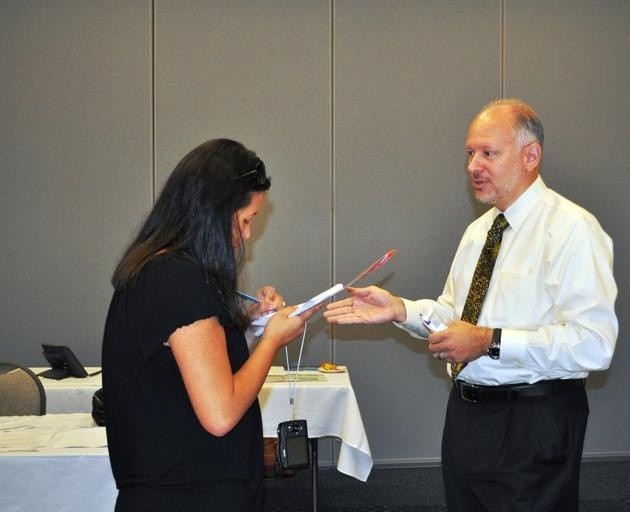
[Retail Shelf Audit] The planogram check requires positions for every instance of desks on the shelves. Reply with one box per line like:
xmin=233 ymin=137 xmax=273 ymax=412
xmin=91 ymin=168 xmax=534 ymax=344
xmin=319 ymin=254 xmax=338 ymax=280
xmin=26 ymin=366 xmax=375 ymax=511
xmin=1 ymin=413 xmax=120 ymax=511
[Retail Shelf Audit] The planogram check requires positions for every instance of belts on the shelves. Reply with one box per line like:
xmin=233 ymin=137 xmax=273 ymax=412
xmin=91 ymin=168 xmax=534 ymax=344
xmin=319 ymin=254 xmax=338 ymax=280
xmin=455 ymin=381 xmax=553 ymax=406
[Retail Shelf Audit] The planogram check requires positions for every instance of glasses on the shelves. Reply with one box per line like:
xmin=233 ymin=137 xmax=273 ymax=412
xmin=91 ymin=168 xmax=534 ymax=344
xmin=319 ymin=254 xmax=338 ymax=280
xmin=234 ymin=160 xmax=267 ymax=185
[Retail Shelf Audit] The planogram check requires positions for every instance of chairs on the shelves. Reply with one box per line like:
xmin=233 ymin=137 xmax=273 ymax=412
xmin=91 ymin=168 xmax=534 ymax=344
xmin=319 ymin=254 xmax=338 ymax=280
xmin=0 ymin=360 xmax=47 ymax=417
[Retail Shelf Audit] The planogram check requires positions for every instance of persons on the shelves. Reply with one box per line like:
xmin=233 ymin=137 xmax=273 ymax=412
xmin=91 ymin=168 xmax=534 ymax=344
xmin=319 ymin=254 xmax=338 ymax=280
xmin=321 ymin=96 xmax=621 ymax=512
xmin=101 ymin=138 xmax=330 ymax=512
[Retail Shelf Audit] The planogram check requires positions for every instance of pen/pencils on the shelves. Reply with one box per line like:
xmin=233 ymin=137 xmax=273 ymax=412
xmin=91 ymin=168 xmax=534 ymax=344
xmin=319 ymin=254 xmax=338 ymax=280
xmin=420 ymin=312 xmax=437 ymax=332
xmin=343 ymin=249 xmax=398 ymax=291
xmin=236 ymin=289 xmax=260 ymax=304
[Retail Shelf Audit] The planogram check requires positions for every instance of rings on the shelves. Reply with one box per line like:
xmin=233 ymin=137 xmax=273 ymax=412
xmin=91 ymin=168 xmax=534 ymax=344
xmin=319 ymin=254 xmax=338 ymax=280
xmin=438 ymin=352 xmax=441 ymax=360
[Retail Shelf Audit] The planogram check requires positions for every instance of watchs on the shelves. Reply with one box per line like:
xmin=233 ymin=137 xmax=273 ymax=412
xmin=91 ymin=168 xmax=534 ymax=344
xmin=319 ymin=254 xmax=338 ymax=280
xmin=486 ymin=328 xmax=502 ymax=361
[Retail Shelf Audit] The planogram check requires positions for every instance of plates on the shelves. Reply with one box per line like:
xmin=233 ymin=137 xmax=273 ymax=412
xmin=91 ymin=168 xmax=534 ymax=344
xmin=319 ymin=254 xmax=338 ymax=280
xmin=318 ymin=366 xmax=346 ymax=372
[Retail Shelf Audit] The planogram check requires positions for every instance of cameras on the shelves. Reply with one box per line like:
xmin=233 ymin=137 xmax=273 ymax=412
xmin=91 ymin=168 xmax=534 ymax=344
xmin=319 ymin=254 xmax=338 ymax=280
xmin=277 ymin=419 xmax=310 ymax=471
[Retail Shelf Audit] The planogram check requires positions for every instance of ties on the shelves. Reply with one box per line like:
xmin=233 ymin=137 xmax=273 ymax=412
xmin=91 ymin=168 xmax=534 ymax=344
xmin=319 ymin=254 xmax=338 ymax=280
xmin=450 ymin=214 xmax=509 ymax=381
